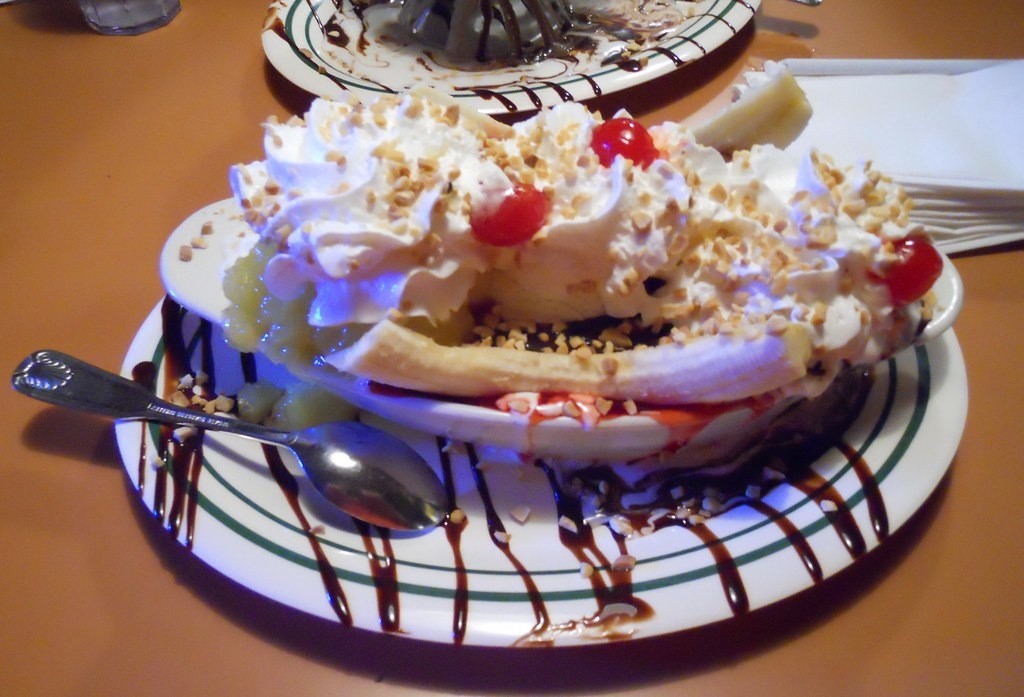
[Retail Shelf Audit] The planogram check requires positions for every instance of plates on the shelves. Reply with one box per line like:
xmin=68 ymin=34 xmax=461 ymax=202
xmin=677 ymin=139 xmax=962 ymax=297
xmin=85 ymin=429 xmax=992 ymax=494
xmin=156 ymin=194 xmax=964 ymax=460
xmin=115 ymin=291 xmax=971 ymax=650
xmin=260 ymin=1 xmax=762 ymax=116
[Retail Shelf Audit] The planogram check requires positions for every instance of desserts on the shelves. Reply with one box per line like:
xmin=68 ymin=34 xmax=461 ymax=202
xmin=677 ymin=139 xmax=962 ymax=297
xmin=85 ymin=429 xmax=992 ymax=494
xmin=223 ymin=85 xmax=944 ymax=419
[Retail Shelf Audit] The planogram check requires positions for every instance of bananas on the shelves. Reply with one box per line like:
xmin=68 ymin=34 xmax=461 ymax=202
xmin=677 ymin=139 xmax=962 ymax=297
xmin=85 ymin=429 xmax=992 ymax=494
xmin=344 ymin=319 xmax=807 ymax=407
xmin=688 ymin=69 xmax=814 ymax=162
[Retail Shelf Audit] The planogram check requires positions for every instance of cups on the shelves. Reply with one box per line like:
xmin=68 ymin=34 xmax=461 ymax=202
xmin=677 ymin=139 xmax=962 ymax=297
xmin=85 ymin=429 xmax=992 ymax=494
xmin=78 ymin=0 xmax=181 ymax=36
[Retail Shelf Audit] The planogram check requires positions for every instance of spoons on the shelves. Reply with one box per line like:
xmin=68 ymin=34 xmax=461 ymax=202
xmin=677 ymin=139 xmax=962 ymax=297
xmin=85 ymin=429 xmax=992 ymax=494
xmin=9 ymin=349 xmax=447 ymax=534
